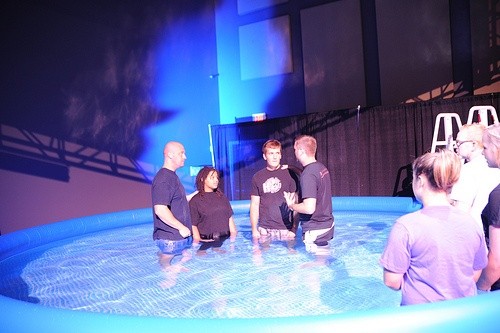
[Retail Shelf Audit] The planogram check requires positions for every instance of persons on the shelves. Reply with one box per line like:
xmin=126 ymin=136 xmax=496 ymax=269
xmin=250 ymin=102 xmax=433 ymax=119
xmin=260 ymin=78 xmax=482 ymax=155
xmin=249 ymin=139 xmax=301 ymax=249
xmin=475 ymin=124 xmax=500 ymax=294
xmin=152 ymin=141 xmax=199 ymax=275
xmin=377 ymin=148 xmax=488 ymax=306
xmin=447 ymin=122 xmax=500 ymax=228
xmin=189 ymin=167 xmax=236 ymax=250
xmin=281 ymin=135 xmax=335 ymax=256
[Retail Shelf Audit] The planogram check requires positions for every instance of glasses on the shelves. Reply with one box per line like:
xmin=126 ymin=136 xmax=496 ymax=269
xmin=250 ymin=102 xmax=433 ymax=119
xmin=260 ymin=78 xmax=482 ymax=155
xmin=455 ymin=140 xmax=473 ymax=147
